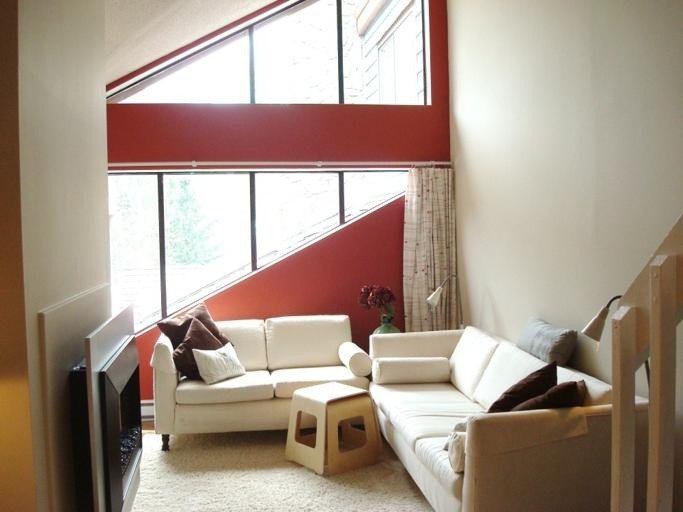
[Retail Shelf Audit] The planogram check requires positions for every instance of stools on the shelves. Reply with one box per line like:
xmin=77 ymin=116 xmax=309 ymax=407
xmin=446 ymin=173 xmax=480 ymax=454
xmin=285 ymin=380 xmax=387 ymax=478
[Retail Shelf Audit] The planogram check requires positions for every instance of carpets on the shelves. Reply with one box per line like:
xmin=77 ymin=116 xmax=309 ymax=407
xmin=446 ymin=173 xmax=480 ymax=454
xmin=128 ymin=427 xmax=436 ymax=512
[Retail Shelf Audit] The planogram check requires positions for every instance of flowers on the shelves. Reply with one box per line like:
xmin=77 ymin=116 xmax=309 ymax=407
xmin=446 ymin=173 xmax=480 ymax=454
xmin=358 ymin=280 xmax=395 ymax=323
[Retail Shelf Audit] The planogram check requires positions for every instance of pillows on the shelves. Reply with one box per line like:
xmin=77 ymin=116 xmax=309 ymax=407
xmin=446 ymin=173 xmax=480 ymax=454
xmin=443 ymin=315 xmax=588 ymax=474
xmin=161 ymin=300 xmax=247 ymax=384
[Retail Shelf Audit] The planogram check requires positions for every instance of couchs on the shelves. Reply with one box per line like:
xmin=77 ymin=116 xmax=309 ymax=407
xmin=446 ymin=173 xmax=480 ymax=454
xmin=148 ymin=315 xmax=372 ymax=451
xmin=368 ymin=312 xmax=648 ymax=512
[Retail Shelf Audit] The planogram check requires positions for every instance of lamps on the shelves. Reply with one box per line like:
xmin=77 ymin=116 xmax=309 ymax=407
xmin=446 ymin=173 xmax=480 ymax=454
xmin=580 ymin=294 xmax=650 ymax=387
xmin=425 ymin=275 xmax=464 ymax=329
